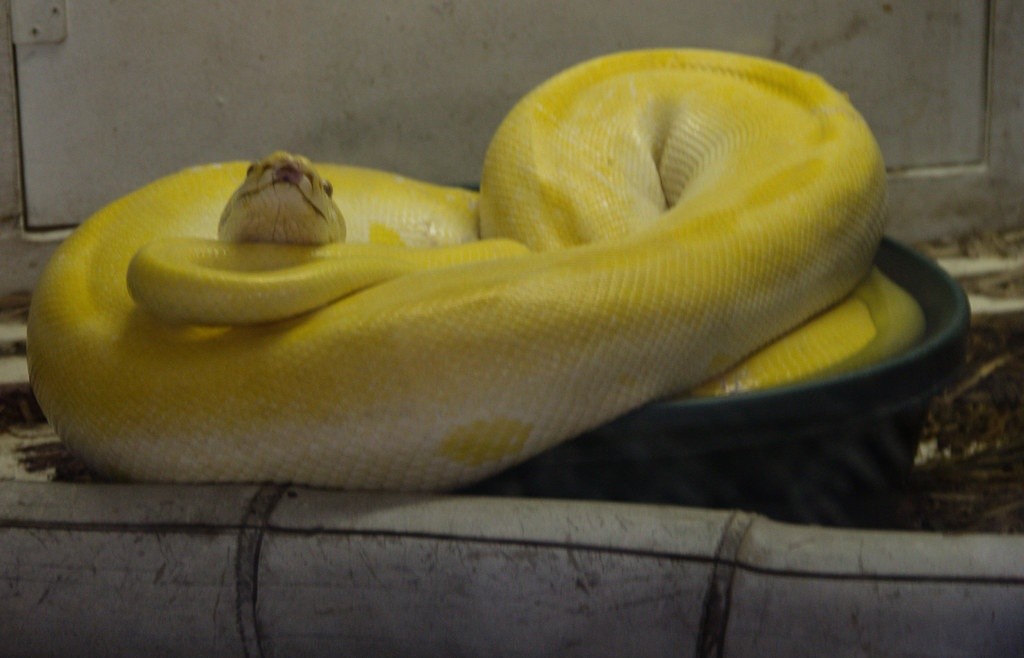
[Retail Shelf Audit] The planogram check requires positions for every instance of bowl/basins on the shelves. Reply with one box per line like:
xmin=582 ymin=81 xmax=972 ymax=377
xmin=570 ymin=224 xmax=971 ymax=519
